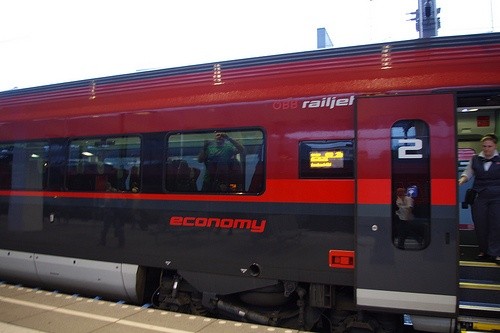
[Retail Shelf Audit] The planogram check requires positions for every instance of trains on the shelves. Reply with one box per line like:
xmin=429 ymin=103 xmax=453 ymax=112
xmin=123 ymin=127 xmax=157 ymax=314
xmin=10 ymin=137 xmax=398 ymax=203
xmin=0 ymin=0 xmax=500 ymax=333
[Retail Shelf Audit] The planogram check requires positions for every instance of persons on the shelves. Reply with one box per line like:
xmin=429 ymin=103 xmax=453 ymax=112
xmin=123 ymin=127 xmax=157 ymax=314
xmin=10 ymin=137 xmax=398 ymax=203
xmin=395 ymin=187 xmax=415 ymax=249
xmin=97 ymin=196 xmax=127 ymax=251
xmin=62 ymin=132 xmax=261 ymax=194
xmin=455 ymin=133 xmax=500 ymax=263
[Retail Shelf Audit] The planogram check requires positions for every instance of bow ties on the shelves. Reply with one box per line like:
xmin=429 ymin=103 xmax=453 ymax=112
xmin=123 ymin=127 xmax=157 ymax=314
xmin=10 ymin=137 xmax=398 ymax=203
xmin=483 ymin=157 xmax=494 ymax=162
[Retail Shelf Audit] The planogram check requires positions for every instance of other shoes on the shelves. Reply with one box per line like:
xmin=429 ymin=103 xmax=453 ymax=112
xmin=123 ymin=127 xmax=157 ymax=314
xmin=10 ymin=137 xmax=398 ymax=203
xmin=478 ymin=251 xmax=486 ymax=257
xmin=396 ymin=245 xmax=404 ymax=249
xmin=492 ymin=256 xmax=500 ymax=264
